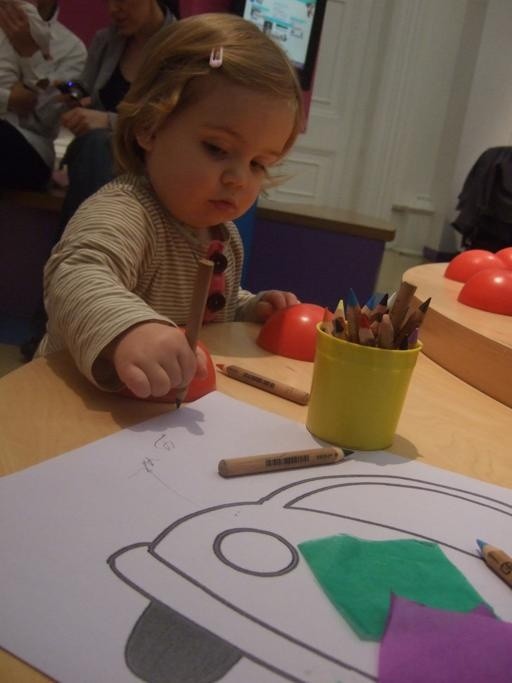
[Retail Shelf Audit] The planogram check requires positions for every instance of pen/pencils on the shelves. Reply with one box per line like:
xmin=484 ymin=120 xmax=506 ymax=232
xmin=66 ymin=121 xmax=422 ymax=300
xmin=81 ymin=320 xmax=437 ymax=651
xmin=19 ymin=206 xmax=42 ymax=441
xmin=477 ymin=541 xmax=512 ymax=587
xmin=323 ymin=282 xmax=432 ymax=351
xmin=174 ymin=258 xmax=307 ymax=408
xmin=218 ymin=447 xmax=356 ymax=478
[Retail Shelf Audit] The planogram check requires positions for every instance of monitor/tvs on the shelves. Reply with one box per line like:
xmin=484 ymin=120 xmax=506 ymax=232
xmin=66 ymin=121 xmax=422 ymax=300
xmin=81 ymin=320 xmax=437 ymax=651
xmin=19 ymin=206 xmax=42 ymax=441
xmin=232 ymin=0 xmax=326 ymax=90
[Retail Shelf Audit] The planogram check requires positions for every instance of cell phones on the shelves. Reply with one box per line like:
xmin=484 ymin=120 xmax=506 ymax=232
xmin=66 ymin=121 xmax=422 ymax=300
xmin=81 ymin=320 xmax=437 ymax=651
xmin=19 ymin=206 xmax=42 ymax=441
xmin=55 ymin=81 xmax=81 ymax=108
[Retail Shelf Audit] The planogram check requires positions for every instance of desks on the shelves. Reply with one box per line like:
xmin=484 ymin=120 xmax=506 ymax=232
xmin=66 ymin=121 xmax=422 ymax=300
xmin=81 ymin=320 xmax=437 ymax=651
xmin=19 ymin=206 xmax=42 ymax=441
xmin=1 ymin=321 xmax=511 ymax=683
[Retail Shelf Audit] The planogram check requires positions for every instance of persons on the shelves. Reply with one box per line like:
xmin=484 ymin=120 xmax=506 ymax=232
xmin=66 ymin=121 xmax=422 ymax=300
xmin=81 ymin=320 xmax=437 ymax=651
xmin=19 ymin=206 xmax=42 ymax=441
xmin=0 ymin=0 xmax=89 ymax=346
xmin=19 ymin=0 xmax=183 ymax=364
xmin=31 ymin=10 xmax=304 ymax=400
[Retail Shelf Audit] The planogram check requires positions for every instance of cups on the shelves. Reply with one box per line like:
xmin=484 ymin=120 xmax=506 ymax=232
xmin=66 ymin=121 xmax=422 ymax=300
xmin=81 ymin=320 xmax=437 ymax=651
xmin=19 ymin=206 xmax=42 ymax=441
xmin=305 ymin=320 xmax=424 ymax=451
